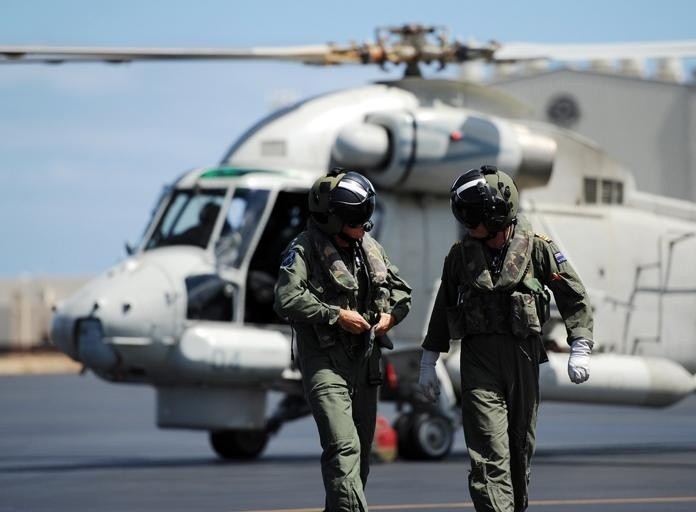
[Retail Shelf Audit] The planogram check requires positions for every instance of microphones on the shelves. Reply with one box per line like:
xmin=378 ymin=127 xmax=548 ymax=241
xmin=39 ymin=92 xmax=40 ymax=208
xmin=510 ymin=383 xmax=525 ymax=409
xmin=363 ymin=219 xmax=374 ymax=232
xmin=463 ymin=219 xmax=497 ymax=250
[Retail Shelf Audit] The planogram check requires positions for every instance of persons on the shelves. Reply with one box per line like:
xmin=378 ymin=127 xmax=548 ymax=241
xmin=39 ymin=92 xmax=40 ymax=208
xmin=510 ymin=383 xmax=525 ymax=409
xmin=274 ymin=167 xmax=412 ymax=512
xmin=198 ymin=202 xmax=231 ymax=236
xmin=418 ymin=166 xmax=593 ymax=512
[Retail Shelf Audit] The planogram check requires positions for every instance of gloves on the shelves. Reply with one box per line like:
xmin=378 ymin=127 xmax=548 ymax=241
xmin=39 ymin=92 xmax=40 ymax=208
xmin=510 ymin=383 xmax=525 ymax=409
xmin=418 ymin=351 xmax=440 ymax=404
xmin=568 ymin=340 xmax=589 ymax=383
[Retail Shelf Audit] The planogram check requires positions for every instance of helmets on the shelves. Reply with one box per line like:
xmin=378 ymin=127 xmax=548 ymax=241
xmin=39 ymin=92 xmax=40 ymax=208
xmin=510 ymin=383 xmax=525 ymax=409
xmin=449 ymin=165 xmax=518 ymax=227
xmin=308 ymin=167 xmax=376 ymax=234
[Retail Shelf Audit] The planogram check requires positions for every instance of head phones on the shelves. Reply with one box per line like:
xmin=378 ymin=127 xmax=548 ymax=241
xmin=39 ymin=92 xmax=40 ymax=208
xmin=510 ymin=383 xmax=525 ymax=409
xmin=482 ymin=185 xmax=509 ymax=224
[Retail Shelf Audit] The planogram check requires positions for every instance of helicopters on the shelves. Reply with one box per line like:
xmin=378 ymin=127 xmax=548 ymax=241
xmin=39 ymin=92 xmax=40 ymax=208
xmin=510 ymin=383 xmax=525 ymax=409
xmin=0 ymin=13 xmax=696 ymax=468
